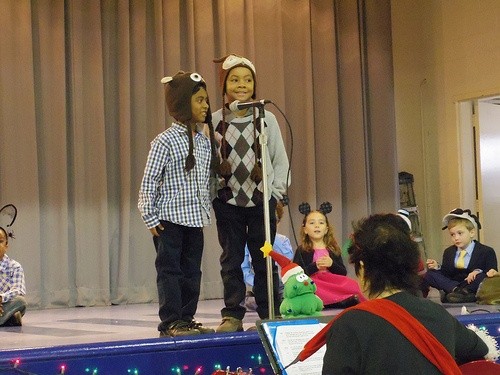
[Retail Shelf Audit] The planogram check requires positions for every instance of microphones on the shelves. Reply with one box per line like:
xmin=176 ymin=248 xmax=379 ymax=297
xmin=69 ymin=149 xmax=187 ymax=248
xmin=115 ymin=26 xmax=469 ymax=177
xmin=229 ymin=99 xmax=272 ymax=112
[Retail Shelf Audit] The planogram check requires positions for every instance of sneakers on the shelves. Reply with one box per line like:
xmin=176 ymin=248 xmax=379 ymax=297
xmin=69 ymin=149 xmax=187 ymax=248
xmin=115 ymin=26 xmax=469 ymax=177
xmin=188 ymin=321 xmax=215 ymax=334
xmin=160 ymin=319 xmax=200 ymax=337
xmin=216 ymin=315 xmax=243 ymax=334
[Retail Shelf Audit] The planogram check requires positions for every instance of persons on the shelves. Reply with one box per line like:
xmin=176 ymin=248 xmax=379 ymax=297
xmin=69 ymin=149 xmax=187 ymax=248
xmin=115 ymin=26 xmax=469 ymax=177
xmin=201 ymin=52 xmax=292 ymax=334
xmin=137 ymin=70 xmax=221 ymax=338
xmin=290 ymin=199 xmax=367 ymax=311
xmin=418 ymin=208 xmax=498 ymax=304
xmin=242 ymin=231 xmax=295 ymax=311
xmin=296 ymin=211 xmax=500 ymax=375
xmin=394 ymin=208 xmax=428 ymax=278
xmin=0 ymin=226 xmax=27 ymax=328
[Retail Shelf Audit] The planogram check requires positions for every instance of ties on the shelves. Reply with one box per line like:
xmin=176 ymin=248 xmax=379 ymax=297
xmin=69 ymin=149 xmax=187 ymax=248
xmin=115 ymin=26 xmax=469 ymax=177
xmin=457 ymin=251 xmax=467 ymax=268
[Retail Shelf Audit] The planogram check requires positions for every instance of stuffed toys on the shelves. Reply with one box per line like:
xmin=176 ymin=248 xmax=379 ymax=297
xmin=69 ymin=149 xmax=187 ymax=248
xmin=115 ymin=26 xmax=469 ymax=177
xmin=259 ymin=240 xmax=324 ymax=319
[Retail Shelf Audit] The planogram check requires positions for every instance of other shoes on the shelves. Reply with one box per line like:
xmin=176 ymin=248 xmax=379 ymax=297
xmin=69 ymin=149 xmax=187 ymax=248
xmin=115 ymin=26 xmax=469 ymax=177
xmin=5 ymin=311 xmax=22 ymax=326
xmin=441 ymin=287 xmax=468 ymax=303
xmin=447 ymin=293 xmax=476 ymax=303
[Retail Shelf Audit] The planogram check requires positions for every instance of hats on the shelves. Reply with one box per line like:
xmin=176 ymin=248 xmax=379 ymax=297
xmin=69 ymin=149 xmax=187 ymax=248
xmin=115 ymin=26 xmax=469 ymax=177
xmin=443 ymin=207 xmax=476 ymax=230
xmin=162 ymin=70 xmax=220 ymax=175
xmin=396 ymin=208 xmax=412 ymax=232
xmin=213 ymin=52 xmax=262 ymax=184
